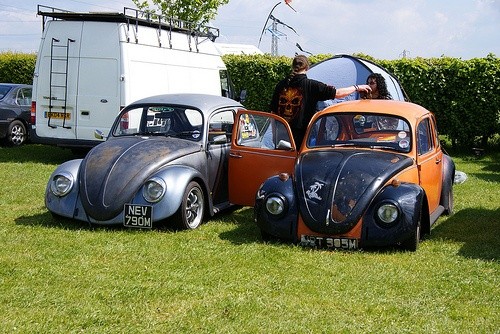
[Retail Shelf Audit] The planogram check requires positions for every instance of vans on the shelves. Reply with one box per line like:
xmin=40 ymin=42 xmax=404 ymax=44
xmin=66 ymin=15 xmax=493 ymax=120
xmin=30 ymin=4 xmax=247 ymax=149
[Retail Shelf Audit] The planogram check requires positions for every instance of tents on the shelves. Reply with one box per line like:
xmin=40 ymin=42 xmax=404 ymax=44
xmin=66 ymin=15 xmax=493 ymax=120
xmin=261 ymin=54 xmax=409 ymax=150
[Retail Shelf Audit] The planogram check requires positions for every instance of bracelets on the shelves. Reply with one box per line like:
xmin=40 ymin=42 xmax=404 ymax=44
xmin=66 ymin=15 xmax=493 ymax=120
xmin=353 ymin=85 xmax=359 ymax=91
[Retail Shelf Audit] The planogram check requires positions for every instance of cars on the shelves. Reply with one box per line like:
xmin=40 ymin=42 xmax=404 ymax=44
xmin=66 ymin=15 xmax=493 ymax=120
xmin=228 ymin=99 xmax=456 ymax=253
xmin=43 ymin=92 xmax=261 ymax=230
xmin=0 ymin=83 xmax=50 ymax=149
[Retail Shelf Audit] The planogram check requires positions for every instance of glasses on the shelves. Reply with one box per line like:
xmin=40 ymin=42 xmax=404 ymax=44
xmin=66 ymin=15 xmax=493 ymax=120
xmin=366 ymin=81 xmax=377 ymax=86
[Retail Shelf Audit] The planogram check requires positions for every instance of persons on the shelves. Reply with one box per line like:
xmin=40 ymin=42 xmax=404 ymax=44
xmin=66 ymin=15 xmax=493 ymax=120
xmin=356 ymin=73 xmax=399 ymax=132
xmin=273 ymin=56 xmax=371 ymax=151
